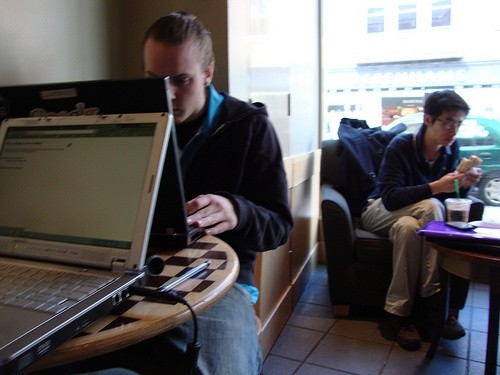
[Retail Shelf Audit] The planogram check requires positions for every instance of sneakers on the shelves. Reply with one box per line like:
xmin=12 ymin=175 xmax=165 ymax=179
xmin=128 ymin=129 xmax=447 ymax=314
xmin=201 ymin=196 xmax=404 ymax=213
xmin=417 ymin=304 xmax=465 ymax=340
xmin=380 ymin=312 xmax=421 ymax=351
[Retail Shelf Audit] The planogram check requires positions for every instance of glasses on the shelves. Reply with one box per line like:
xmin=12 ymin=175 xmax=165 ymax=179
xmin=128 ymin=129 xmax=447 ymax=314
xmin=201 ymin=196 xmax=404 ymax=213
xmin=435 ymin=118 xmax=463 ymax=128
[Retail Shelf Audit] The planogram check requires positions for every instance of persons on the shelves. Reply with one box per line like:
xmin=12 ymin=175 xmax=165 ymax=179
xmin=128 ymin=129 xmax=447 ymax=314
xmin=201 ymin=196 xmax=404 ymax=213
xmin=93 ymin=11 xmax=294 ymax=375
xmin=359 ymin=88 xmax=483 ymax=350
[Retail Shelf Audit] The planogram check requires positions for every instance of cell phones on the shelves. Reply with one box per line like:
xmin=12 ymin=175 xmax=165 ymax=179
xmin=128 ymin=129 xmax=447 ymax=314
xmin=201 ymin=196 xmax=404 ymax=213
xmin=445 ymin=221 xmax=477 ymax=231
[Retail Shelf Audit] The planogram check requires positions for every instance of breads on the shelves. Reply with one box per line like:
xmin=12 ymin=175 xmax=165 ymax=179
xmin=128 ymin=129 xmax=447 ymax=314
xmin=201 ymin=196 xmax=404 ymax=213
xmin=457 ymin=155 xmax=483 ymax=173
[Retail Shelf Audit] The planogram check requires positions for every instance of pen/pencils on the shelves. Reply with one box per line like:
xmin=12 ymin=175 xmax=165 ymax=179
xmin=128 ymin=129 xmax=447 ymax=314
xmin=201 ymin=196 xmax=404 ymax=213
xmin=454 ymin=170 xmax=460 ymax=199
xmin=158 ymin=261 xmax=211 ymax=293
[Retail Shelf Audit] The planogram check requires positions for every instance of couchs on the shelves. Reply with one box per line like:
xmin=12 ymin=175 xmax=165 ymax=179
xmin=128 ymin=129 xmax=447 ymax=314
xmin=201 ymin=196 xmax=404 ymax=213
xmin=318 ymin=140 xmax=484 ymax=321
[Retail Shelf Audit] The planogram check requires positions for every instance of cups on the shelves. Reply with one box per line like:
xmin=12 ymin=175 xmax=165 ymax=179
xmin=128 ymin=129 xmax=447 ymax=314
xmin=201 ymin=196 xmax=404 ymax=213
xmin=445 ymin=198 xmax=472 ymax=224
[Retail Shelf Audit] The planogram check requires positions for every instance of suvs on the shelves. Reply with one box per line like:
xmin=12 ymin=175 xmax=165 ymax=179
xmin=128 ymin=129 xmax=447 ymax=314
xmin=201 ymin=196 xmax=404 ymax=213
xmin=380 ymin=114 xmax=500 ymax=205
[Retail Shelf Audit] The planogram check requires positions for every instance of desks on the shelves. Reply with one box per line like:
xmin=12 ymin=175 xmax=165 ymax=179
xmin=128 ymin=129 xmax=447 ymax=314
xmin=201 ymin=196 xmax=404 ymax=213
xmin=21 ymin=234 xmax=239 ymax=375
xmin=426 ymin=207 xmax=500 ymax=375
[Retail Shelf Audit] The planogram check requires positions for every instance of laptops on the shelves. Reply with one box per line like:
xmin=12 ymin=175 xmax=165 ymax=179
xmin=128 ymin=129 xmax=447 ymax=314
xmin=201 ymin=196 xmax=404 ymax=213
xmin=0 ymin=76 xmax=206 ymax=249
xmin=0 ymin=112 xmax=174 ymax=375
